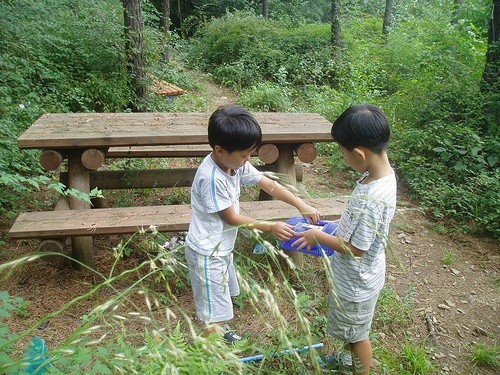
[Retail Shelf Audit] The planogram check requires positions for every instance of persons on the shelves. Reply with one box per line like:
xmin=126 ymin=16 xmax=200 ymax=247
xmin=183 ymin=105 xmax=323 ymax=342
xmin=291 ymin=104 xmax=398 ymax=375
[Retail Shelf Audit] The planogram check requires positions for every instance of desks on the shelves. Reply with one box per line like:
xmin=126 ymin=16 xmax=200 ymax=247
xmin=18 ymin=113 xmax=335 ymax=272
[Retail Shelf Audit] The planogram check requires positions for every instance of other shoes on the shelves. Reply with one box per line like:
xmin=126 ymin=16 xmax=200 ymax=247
xmin=220 ymin=325 xmax=244 ymax=345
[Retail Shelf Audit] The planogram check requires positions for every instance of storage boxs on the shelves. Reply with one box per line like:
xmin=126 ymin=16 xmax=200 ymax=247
xmin=278 ymin=216 xmax=339 ymax=257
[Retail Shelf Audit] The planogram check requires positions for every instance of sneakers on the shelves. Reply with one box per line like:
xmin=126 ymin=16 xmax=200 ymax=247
xmin=316 ymin=354 xmax=353 ymax=374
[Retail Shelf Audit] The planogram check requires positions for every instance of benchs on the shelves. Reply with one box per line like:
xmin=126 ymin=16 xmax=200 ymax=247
xmin=9 ymin=197 xmax=342 ymax=238
xmin=105 ymin=144 xmax=297 ymax=158
xmin=145 ymin=73 xmax=184 ymax=103
